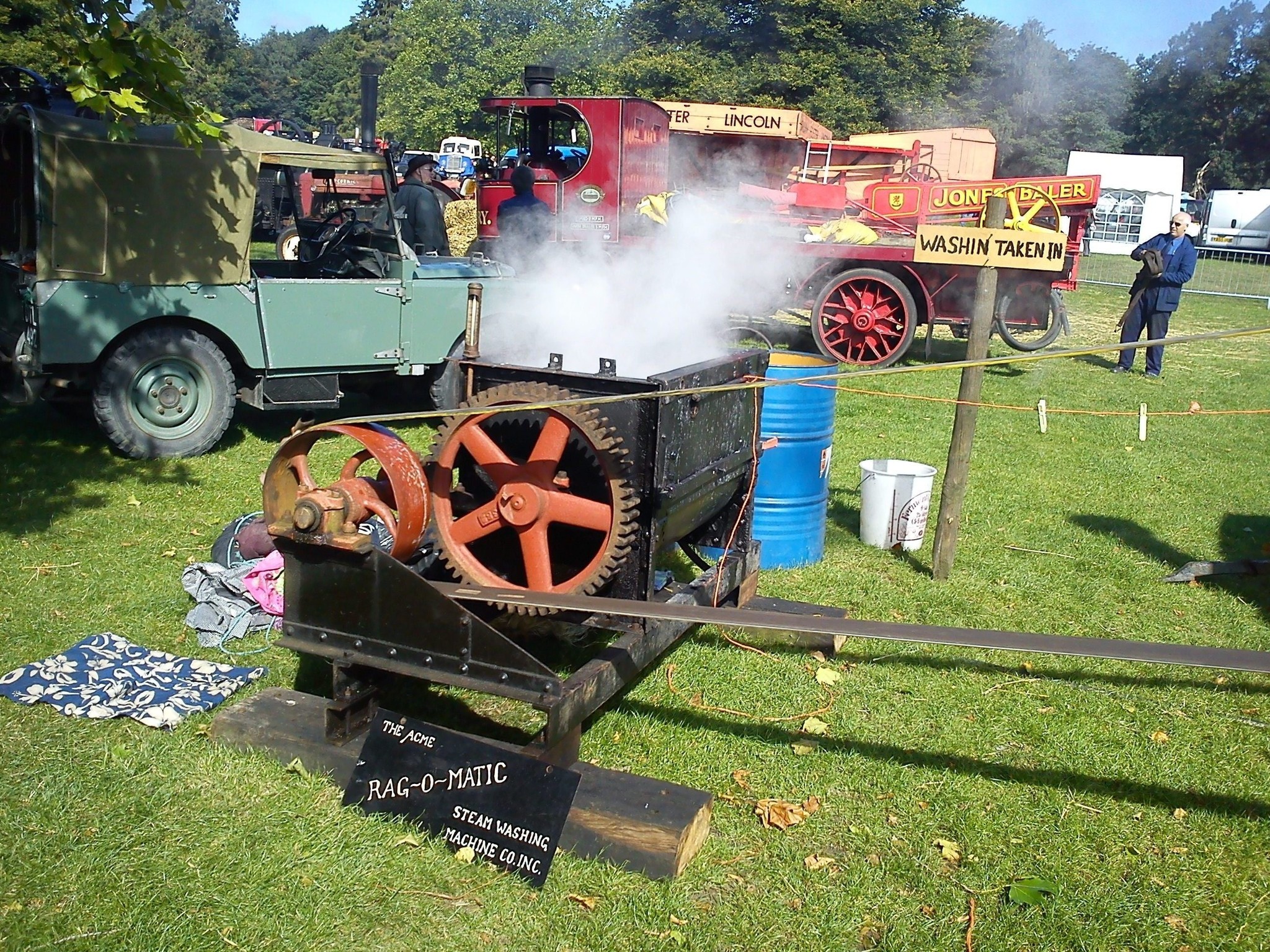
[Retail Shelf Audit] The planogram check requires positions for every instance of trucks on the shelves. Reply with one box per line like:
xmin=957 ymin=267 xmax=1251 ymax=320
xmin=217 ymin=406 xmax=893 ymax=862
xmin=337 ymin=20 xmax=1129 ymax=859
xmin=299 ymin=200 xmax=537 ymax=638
xmin=230 ymin=116 xmax=590 ymax=267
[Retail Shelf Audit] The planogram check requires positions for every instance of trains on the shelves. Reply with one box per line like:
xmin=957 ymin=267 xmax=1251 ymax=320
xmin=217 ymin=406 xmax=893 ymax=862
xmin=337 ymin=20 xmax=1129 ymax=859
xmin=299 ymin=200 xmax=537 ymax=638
xmin=462 ymin=64 xmax=1100 ymax=371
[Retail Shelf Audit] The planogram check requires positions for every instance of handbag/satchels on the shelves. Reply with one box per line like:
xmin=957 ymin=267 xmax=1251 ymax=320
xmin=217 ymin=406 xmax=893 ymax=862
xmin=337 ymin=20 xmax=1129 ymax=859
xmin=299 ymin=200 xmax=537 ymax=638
xmin=1142 ymin=248 xmax=1163 ymax=275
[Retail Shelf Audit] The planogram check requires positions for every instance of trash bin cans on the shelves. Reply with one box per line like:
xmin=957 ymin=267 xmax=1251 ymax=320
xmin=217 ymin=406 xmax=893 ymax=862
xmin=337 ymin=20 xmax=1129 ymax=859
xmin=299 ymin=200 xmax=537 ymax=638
xmin=694 ymin=349 xmax=840 ymax=570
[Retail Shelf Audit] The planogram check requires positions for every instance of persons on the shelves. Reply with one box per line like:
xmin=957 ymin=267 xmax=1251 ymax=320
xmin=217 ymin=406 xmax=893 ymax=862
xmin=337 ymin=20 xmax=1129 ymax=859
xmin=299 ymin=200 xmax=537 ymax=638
xmin=496 ymin=165 xmax=554 ymax=260
xmin=1082 ymin=209 xmax=1097 ymax=257
xmin=476 ymin=154 xmax=497 ymax=179
xmin=388 ymin=153 xmax=452 ymax=257
xmin=1111 ymin=212 xmax=1198 ymax=376
xmin=1181 ymin=208 xmax=1201 ymax=247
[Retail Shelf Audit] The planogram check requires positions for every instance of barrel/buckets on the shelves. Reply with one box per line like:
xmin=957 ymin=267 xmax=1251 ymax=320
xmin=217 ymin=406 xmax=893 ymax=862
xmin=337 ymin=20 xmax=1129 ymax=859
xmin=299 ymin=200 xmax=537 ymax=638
xmin=752 ymin=349 xmax=840 ymax=570
xmin=855 ymin=459 xmax=938 ymax=552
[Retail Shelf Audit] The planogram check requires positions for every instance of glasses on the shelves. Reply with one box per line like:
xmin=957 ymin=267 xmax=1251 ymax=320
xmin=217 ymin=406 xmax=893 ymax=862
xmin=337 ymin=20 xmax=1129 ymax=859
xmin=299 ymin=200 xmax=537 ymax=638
xmin=420 ymin=166 xmax=433 ymax=172
xmin=1170 ymin=220 xmax=1181 ymax=227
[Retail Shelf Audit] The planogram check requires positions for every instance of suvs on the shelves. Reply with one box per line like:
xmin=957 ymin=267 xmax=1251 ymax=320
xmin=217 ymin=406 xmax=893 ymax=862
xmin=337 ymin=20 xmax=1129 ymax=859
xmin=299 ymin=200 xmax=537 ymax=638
xmin=0 ymin=101 xmax=524 ymax=464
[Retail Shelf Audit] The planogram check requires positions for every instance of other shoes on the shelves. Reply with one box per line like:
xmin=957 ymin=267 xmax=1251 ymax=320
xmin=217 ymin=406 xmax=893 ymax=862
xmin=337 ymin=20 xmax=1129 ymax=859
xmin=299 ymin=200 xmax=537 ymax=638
xmin=1145 ymin=373 xmax=1156 ymax=379
xmin=1111 ymin=365 xmax=1129 ymax=374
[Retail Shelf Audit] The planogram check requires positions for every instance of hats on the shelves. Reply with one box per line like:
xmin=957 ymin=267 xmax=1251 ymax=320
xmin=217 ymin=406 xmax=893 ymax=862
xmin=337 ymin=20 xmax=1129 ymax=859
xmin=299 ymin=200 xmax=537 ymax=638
xmin=403 ymin=153 xmax=440 ymax=179
